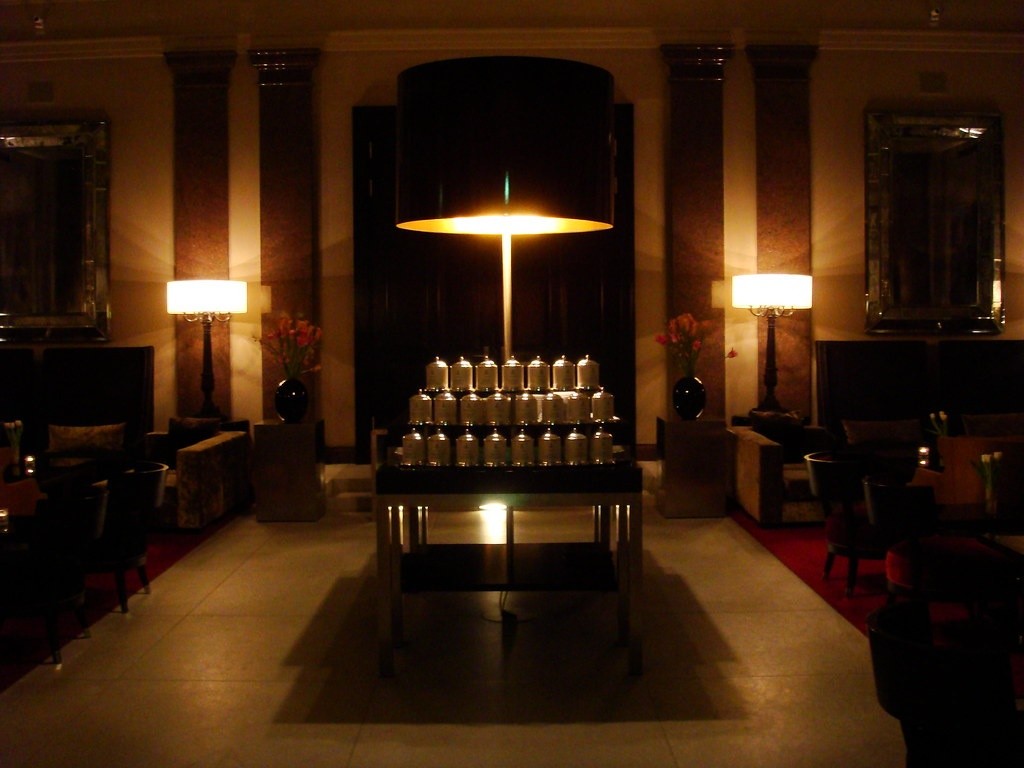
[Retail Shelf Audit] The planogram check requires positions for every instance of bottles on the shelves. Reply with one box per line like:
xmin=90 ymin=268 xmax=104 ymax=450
xmin=407 ymin=389 xmax=615 ymax=426
xmin=400 ymin=427 xmax=616 ymax=467
xmin=426 ymin=355 xmax=600 ymax=391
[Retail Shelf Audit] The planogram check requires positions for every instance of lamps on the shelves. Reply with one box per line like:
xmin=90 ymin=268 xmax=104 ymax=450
xmin=390 ymin=53 xmax=617 ymax=237
xmin=166 ymin=280 xmax=247 ymax=415
xmin=732 ymin=273 xmax=815 ymax=427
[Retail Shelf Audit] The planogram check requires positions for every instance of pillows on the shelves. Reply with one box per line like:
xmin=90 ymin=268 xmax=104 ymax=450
xmin=47 ymin=423 xmax=127 ymax=449
xmin=168 ymin=416 xmax=222 ymax=448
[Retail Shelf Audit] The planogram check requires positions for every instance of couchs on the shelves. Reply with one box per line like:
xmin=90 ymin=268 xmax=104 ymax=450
xmin=142 ymin=431 xmax=247 ymax=531
xmin=727 ymin=426 xmax=861 ymax=528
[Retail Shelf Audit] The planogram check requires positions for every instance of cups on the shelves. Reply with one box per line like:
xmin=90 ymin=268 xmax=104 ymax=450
xmin=0 ymin=507 xmax=9 ymax=534
xmin=24 ymin=455 xmax=36 ymax=477
xmin=917 ymin=446 xmax=930 ymax=468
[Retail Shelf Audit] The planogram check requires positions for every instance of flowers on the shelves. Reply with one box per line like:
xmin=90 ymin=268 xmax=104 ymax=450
xmin=2 ymin=420 xmax=25 ymax=480
xmin=974 ymin=451 xmax=1004 ymax=515
xmin=929 ymin=411 xmax=948 ymax=437
xmin=654 ymin=316 xmax=715 ymax=373
xmin=253 ymin=317 xmax=326 ymax=379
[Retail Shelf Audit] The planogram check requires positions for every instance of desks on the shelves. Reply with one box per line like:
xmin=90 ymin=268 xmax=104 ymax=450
xmin=254 ymin=418 xmax=327 ymax=523
xmin=665 ymin=420 xmax=726 ymax=517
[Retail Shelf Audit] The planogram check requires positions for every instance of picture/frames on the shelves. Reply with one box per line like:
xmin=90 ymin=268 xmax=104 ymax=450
xmin=862 ymin=107 xmax=1007 ymax=336
xmin=0 ymin=115 xmax=112 ymax=344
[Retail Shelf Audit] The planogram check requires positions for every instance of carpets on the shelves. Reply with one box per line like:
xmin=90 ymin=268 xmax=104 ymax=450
xmin=0 ymin=504 xmax=241 ymax=691
xmin=726 ymin=509 xmax=1023 ymax=699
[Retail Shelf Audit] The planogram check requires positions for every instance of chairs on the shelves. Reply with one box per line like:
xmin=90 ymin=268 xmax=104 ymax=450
xmin=79 ymin=461 xmax=169 ymax=614
xmin=868 ymin=604 xmax=1024 ymax=768
xmin=1 ymin=480 xmax=112 ymax=666
xmin=863 ymin=480 xmax=1023 ymax=648
xmin=805 ymin=450 xmax=915 ymax=594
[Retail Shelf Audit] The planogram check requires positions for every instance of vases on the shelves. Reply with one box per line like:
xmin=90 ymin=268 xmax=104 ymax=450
xmin=673 ymin=367 xmax=708 ymax=420
xmin=272 ymin=375 xmax=310 ymax=423
